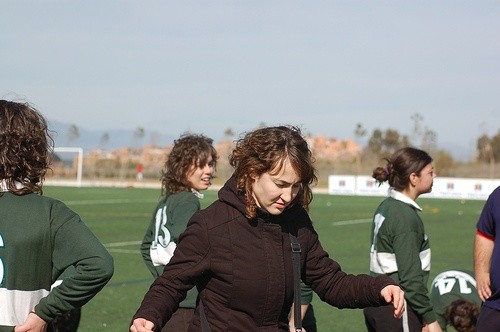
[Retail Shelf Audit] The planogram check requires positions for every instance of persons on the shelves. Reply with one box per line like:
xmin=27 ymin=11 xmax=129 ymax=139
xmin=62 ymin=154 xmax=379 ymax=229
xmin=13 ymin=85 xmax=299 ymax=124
xmin=287 ymin=276 xmax=318 ymax=332
xmin=364 ymin=147 xmax=444 ymax=332
xmin=141 ymin=134 xmax=219 ymax=332
xmin=127 ymin=124 xmax=405 ymax=332
xmin=0 ymin=99 xmax=114 ymax=332
xmin=474 ymin=185 xmax=500 ymax=332
xmin=429 ymin=269 xmax=476 ymax=332
